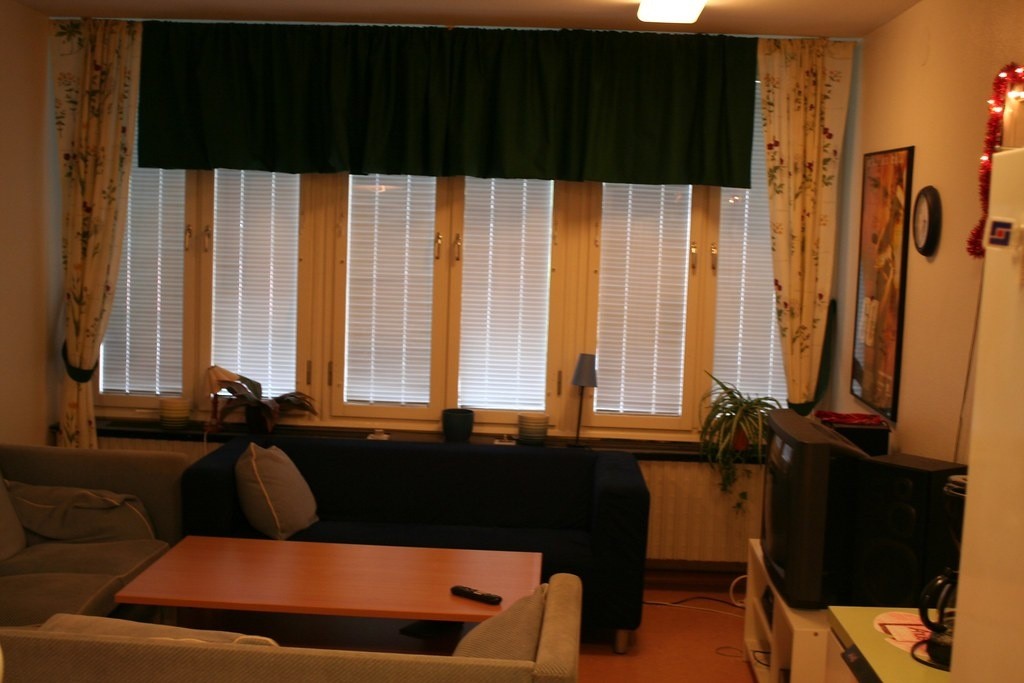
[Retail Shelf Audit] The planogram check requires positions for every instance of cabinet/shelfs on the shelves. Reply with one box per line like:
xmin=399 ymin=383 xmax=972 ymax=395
xmin=743 ymin=538 xmax=831 ymax=683
xmin=826 ymin=605 xmax=953 ymax=683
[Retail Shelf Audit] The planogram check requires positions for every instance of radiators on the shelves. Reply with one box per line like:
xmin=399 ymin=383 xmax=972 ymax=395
xmin=636 ymin=458 xmax=766 ymax=566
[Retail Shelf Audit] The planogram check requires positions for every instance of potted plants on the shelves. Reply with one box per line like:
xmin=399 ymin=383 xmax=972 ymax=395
xmin=219 ymin=375 xmax=320 ymax=434
xmin=696 ymin=369 xmax=783 ymax=514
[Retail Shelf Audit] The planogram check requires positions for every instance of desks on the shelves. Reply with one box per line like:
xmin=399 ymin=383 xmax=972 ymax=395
xmin=115 ymin=535 xmax=543 ymax=625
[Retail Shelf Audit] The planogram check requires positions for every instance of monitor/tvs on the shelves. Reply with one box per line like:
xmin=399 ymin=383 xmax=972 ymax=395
xmin=760 ymin=407 xmax=874 ymax=610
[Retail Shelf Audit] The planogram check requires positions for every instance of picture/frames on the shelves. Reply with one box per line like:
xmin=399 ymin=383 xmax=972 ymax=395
xmin=850 ymin=145 xmax=914 ymax=423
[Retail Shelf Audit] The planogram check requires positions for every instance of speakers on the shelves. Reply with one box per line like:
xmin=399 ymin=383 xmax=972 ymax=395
xmin=856 ymin=453 xmax=968 ymax=610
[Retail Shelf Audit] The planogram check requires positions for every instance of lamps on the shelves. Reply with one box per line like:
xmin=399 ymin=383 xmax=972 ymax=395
xmin=568 ymin=353 xmax=597 ymax=451
xmin=202 ymin=366 xmax=241 ymax=433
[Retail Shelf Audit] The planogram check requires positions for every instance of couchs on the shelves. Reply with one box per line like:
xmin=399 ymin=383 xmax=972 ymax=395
xmin=0 ymin=442 xmax=189 ymax=631
xmin=179 ymin=436 xmax=650 ymax=654
xmin=0 ymin=573 xmax=583 ymax=683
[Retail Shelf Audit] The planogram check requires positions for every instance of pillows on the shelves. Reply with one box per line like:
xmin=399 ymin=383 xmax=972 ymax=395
xmin=38 ymin=612 xmax=281 ymax=647
xmin=452 ymin=583 xmax=548 ymax=661
xmin=235 ymin=443 xmax=320 ymax=542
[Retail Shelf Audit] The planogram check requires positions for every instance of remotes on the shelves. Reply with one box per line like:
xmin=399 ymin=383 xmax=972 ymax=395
xmin=451 ymin=586 xmax=502 ymax=604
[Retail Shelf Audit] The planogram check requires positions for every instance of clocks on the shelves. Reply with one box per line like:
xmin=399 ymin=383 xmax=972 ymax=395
xmin=912 ymin=186 xmax=940 ymax=256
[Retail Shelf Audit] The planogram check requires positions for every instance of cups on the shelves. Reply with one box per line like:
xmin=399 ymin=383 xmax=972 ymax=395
xmin=516 ymin=412 xmax=549 ymax=447
xmin=442 ymin=409 xmax=473 ymax=442
